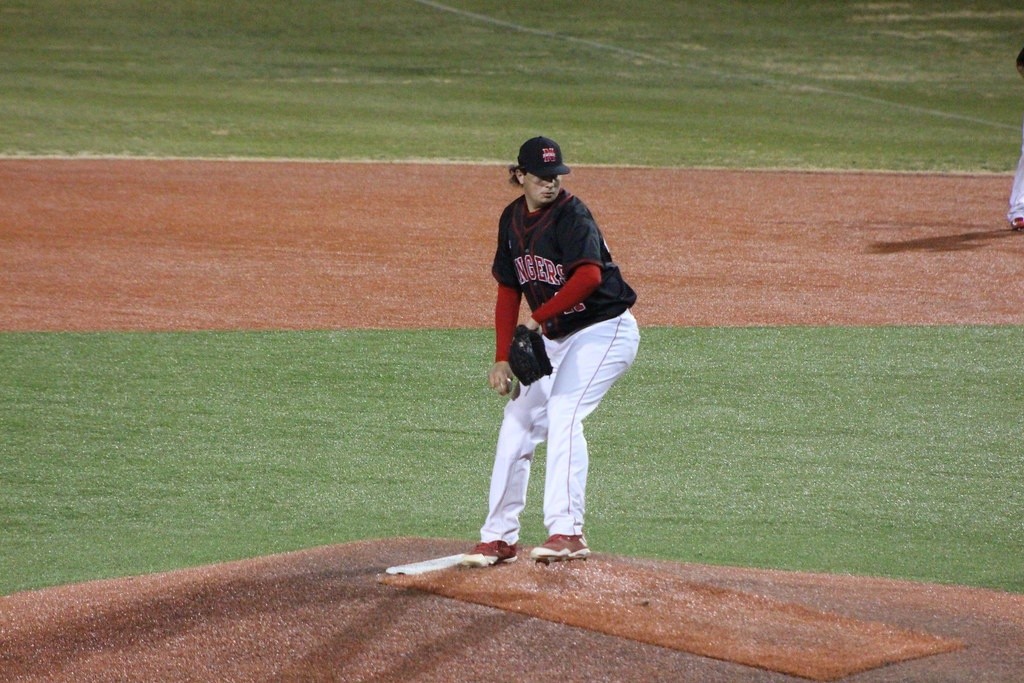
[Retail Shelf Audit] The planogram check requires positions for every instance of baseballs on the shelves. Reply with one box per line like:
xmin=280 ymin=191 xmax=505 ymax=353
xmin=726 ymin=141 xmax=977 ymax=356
xmin=500 ymin=378 xmax=512 ymax=392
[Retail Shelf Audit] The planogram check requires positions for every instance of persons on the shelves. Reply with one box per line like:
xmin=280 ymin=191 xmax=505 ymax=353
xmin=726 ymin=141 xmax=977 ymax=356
xmin=470 ymin=136 xmax=641 ymax=562
xmin=1006 ymin=49 xmax=1024 ymax=230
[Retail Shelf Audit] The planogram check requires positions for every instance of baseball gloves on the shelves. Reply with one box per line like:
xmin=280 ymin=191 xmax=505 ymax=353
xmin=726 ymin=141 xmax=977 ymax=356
xmin=508 ymin=324 xmax=554 ymax=398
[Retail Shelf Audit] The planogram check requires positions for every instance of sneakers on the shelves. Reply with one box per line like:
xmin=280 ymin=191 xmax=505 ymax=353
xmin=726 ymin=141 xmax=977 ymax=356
xmin=461 ymin=540 xmax=517 ymax=567
xmin=1012 ymin=216 xmax=1024 ymax=230
xmin=530 ymin=533 xmax=591 ymax=562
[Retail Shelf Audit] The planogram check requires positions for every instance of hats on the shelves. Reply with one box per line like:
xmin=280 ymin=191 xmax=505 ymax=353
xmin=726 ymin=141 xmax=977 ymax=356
xmin=517 ymin=136 xmax=570 ymax=178
